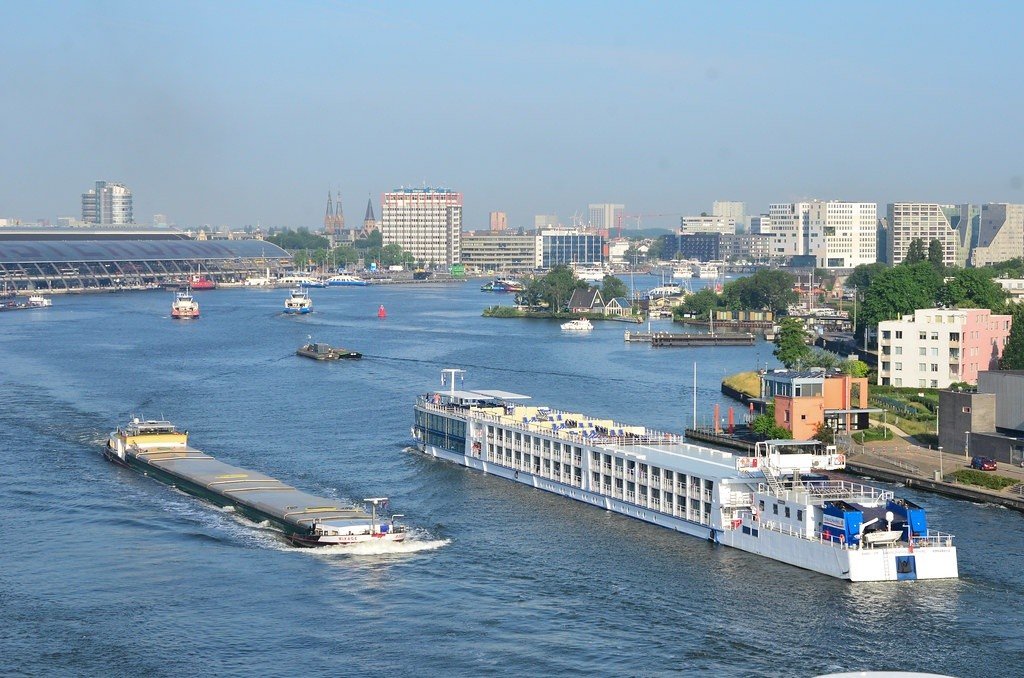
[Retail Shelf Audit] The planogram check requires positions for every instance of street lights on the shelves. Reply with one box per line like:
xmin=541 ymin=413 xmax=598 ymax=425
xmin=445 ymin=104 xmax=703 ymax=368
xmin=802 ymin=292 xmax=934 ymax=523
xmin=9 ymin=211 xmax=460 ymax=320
xmin=937 ymin=446 xmax=943 ymax=479
xmin=884 ymin=408 xmax=888 ymax=439
xmin=964 ymin=431 xmax=970 ymax=461
xmin=935 ymin=406 xmax=939 ymax=444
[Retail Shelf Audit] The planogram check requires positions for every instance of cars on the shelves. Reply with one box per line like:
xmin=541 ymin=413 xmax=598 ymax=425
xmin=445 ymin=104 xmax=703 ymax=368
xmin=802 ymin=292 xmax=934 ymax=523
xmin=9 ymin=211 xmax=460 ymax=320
xmin=970 ymin=455 xmax=998 ymax=471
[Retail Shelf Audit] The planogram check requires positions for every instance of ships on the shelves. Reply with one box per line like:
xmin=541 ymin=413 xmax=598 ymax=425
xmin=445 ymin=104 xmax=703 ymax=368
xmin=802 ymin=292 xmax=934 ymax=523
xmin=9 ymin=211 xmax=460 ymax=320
xmin=409 ymin=367 xmax=961 ymax=584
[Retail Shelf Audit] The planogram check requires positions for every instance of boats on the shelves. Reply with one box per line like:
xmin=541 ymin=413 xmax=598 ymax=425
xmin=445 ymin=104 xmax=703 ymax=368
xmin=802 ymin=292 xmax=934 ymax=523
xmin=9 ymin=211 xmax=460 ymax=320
xmin=0 ymin=299 xmax=25 ymax=310
xmin=642 ymin=270 xmax=687 ymax=300
xmin=864 ymin=530 xmax=904 ymax=545
xmin=104 ymin=411 xmax=410 ymax=550
xmin=170 ymin=286 xmax=200 ymax=319
xmin=27 ymin=286 xmax=53 ymax=308
xmin=296 ymin=334 xmax=362 ymax=361
xmin=187 ymin=263 xmax=216 ymax=291
xmin=481 ymin=275 xmax=527 ymax=293
xmin=323 ymin=274 xmax=370 ymax=286
xmin=690 ymin=262 xmax=719 ymax=278
xmin=559 ymin=316 xmax=594 ymax=330
xmin=649 ymin=263 xmax=694 ymax=278
xmin=279 ymin=277 xmax=326 ymax=289
xmin=282 ymin=278 xmax=314 ymax=314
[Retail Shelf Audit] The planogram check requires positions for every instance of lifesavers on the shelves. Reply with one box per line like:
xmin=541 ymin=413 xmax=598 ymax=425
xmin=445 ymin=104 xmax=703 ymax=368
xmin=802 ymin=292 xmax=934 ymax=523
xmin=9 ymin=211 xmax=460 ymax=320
xmin=838 ymin=455 xmax=844 ymax=462
xmin=840 ymin=534 xmax=844 ymax=543
